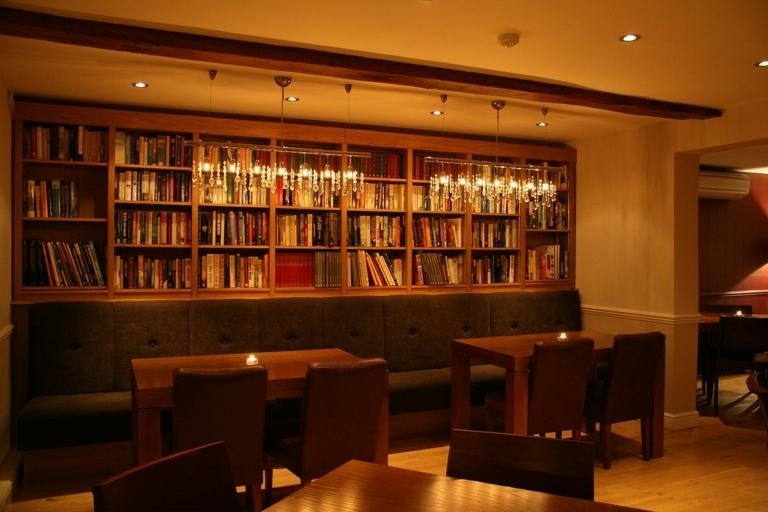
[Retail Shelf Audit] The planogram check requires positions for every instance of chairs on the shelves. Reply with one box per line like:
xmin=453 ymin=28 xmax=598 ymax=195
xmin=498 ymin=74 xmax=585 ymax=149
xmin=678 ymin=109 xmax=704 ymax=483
xmin=165 ymin=359 xmax=274 ymax=510
xmin=703 ymin=311 xmax=762 ymax=413
xmin=483 ymin=334 xmax=591 ymax=444
xmin=88 ymin=439 xmax=245 ymax=511
xmin=444 ymin=426 xmax=594 ymax=501
xmin=702 ymin=302 xmax=755 ymax=316
xmin=258 ymin=344 xmax=389 ymax=488
xmin=569 ymin=332 xmax=665 ymax=471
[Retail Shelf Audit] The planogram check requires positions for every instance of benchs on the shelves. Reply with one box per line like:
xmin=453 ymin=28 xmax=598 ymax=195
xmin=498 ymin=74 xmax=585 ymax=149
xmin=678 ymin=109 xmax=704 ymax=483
xmin=13 ymin=286 xmax=588 ymax=491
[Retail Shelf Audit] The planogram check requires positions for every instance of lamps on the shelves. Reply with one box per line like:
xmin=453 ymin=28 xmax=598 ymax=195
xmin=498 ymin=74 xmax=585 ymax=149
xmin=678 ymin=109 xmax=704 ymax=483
xmin=183 ymin=67 xmax=366 ymax=199
xmin=426 ymin=92 xmax=559 ymax=208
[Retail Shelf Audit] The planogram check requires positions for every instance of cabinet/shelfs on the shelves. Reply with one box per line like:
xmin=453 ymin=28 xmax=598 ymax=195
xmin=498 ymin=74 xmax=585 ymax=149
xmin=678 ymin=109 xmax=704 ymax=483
xmin=11 ymin=96 xmax=578 ymax=305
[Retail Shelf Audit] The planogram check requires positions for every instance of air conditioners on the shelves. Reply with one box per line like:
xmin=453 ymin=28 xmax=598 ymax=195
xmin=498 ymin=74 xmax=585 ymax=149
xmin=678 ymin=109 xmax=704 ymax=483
xmin=694 ymin=164 xmax=753 ymax=200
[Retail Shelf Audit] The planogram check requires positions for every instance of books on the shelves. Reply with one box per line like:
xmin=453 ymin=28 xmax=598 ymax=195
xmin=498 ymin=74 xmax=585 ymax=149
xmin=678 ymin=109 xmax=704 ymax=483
xmin=346 ymin=150 xmax=406 ymax=212
xmin=410 ymin=152 xmax=466 ymax=211
xmin=472 ymin=158 xmax=519 ymax=215
xmin=113 ymin=210 xmax=193 ymax=287
xmin=113 ymin=131 xmax=192 ymax=204
xmin=25 ymin=178 xmax=80 ymax=219
xmin=274 ymin=213 xmax=341 ymax=286
xmin=24 ymin=126 xmax=110 ymax=164
xmin=525 ymin=160 xmax=567 ymax=230
xmin=276 ymin=145 xmax=341 ymax=208
xmin=346 ymin=215 xmax=405 ymax=286
xmin=202 ymin=143 xmax=272 ymax=206
xmin=24 ymin=238 xmax=107 ymax=286
xmin=413 ymin=216 xmax=465 ymax=286
xmin=198 ymin=208 xmax=269 ymax=287
xmin=471 ymin=218 xmax=517 ymax=284
xmin=526 ymin=243 xmax=561 ymax=281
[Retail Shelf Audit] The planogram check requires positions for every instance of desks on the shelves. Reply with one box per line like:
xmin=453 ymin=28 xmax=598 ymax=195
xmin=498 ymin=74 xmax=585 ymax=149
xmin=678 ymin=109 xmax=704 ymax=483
xmin=696 ymin=312 xmax=767 ymax=373
xmin=435 ymin=326 xmax=672 ymax=462
xmin=248 ymin=457 xmax=653 ymax=511
xmin=109 ymin=347 xmax=392 ymax=478
